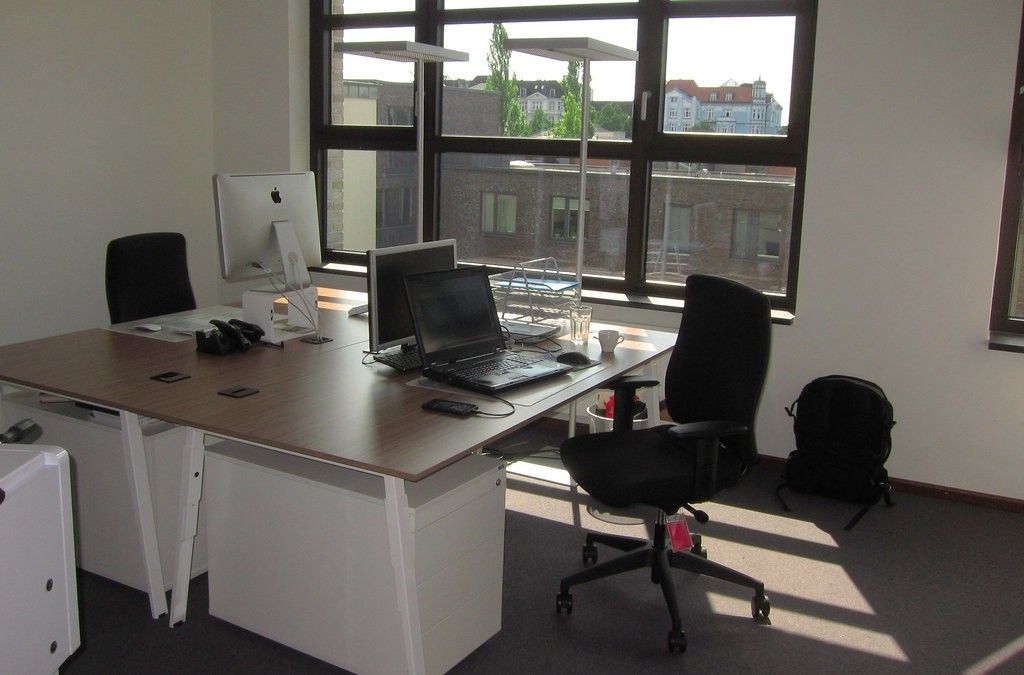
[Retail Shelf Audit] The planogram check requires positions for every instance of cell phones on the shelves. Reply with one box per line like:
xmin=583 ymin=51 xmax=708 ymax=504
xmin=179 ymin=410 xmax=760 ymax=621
xmin=422 ymin=399 xmax=478 ymax=416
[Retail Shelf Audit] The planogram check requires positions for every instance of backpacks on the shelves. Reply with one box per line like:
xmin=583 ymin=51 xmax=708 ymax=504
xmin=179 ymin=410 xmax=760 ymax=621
xmin=787 ymin=374 xmax=894 ymax=505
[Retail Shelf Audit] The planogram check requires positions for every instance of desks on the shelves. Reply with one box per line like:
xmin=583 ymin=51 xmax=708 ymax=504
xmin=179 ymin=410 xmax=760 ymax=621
xmin=0 ymin=286 xmax=679 ymax=675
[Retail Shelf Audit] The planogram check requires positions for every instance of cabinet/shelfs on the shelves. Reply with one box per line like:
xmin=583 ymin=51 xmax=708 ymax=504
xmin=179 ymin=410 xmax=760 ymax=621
xmin=204 ymin=441 xmax=507 ymax=675
xmin=0 ymin=384 xmax=226 ymax=593
xmin=0 ymin=443 xmax=80 ymax=675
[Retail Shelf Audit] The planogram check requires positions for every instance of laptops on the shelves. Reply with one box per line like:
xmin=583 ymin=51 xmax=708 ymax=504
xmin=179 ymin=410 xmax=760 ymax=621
xmin=402 ymin=264 xmax=574 ymax=395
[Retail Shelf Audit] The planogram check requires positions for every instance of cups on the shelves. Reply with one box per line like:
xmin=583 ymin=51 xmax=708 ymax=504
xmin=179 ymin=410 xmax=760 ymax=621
xmin=569 ymin=305 xmax=592 ymax=344
xmin=599 ymin=330 xmax=624 ymax=353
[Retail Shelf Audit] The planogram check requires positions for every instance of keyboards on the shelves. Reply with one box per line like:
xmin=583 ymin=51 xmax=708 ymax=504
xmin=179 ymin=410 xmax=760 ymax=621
xmin=374 ymin=350 xmax=423 ymax=371
xmin=348 ymin=305 xmax=369 ymax=316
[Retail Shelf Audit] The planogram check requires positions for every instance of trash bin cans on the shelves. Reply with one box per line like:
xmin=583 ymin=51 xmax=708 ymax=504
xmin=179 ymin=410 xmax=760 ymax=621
xmin=586 ymin=401 xmax=650 ymax=433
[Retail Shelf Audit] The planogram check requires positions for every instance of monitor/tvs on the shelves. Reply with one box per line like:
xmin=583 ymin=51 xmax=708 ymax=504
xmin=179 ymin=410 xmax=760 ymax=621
xmin=367 ymin=239 xmax=458 ymax=352
xmin=212 ymin=171 xmax=321 ymax=293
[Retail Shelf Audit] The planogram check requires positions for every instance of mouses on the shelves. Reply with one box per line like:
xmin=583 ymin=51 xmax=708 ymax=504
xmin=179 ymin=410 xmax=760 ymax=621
xmin=556 ymin=352 xmax=591 ymax=365
xmin=134 ymin=323 xmax=162 ymax=331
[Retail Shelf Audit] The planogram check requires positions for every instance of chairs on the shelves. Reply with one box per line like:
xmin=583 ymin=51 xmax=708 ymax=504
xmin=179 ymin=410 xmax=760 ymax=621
xmin=105 ymin=232 xmax=198 ymax=325
xmin=556 ymin=274 xmax=772 ymax=655
